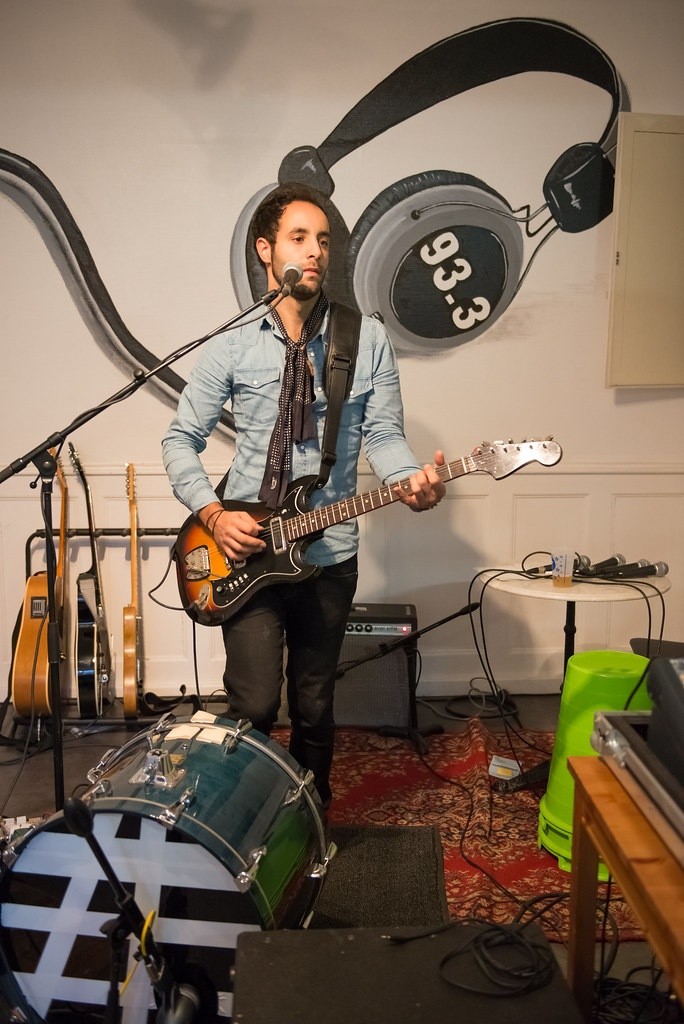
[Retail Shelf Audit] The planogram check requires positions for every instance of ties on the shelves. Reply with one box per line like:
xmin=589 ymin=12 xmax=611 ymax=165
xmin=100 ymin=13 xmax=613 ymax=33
xmin=257 ymin=292 xmax=328 ymax=512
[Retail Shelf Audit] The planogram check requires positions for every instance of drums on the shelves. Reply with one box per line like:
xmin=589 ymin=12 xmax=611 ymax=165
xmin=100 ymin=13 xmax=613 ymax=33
xmin=0 ymin=709 xmax=338 ymax=1024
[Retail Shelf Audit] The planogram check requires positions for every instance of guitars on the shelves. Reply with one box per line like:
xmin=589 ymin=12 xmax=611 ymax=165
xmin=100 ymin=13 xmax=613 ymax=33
xmin=122 ymin=461 xmax=144 ymax=719
xmin=170 ymin=435 xmax=563 ymax=626
xmin=10 ymin=447 xmax=70 ymax=718
xmin=68 ymin=443 xmax=116 ymax=719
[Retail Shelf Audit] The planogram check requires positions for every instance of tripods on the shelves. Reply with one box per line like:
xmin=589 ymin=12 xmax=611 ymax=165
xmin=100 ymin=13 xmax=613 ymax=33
xmin=336 ymin=601 xmax=481 ymax=754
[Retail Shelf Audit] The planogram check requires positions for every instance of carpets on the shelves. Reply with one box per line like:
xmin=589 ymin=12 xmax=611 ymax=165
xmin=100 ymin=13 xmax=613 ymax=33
xmin=260 ymin=709 xmax=650 ymax=944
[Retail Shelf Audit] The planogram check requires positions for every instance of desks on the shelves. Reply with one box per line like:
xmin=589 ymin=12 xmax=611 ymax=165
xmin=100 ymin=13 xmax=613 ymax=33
xmin=565 ymin=756 xmax=684 ymax=1009
xmin=478 ymin=562 xmax=673 ymax=794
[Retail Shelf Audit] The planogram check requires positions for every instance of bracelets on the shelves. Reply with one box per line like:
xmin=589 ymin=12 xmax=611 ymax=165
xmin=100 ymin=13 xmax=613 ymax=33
xmin=205 ymin=508 xmax=225 ymax=527
xmin=212 ymin=510 xmax=224 ymax=535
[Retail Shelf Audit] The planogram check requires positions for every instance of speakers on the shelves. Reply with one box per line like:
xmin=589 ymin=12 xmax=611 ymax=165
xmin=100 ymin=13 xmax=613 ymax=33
xmin=272 ymin=604 xmax=417 ymax=730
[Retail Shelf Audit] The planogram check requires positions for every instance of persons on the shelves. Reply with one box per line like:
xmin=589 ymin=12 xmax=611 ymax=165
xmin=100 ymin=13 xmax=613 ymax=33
xmin=160 ymin=190 xmax=448 ymax=812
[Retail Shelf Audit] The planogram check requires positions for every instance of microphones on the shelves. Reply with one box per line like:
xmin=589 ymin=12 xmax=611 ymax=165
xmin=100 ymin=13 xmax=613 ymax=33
xmin=499 ymin=689 xmax=516 ymax=708
xmin=527 ymin=554 xmax=669 ymax=579
xmin=154 ymin=959 xmax=219 ymax=1024
xmin=282 ymin=262 xmax=303 ymax=298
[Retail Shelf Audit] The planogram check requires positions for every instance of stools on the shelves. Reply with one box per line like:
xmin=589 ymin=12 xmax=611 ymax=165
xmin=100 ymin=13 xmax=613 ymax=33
xmin=536 ymin=650 xmax=655 ymax=884
